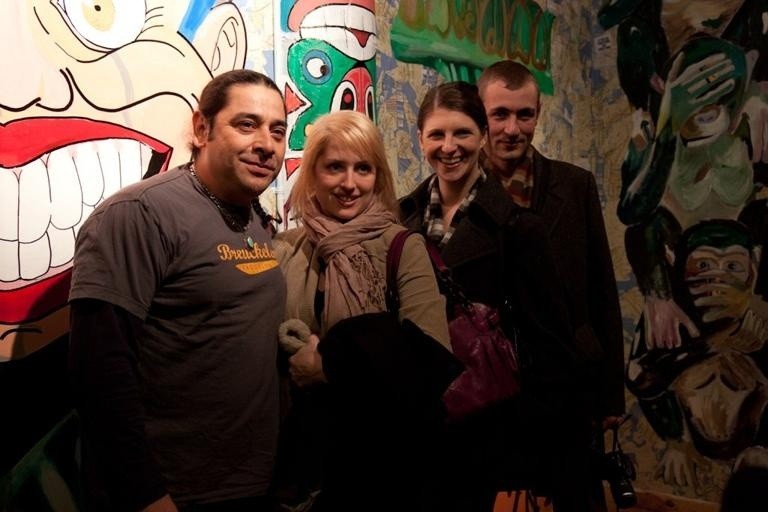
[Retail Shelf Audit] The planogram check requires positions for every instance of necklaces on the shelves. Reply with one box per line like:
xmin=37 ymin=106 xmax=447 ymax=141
xmin=187 ymin=161 xmax=256 ymax=251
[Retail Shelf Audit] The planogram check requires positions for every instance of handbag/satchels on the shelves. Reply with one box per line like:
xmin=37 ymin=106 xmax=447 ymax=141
xmin=385 ymin=225 xmax=525 ymax=423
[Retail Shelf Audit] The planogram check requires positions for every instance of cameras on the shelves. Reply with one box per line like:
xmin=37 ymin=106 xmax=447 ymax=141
xmin=605 ymin=451 xmax=636 ymax=509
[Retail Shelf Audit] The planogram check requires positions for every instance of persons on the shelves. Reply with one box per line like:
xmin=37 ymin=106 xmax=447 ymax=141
xmin=269 ymin=108 xmax=470 ymax=509
xmin=66 ymin=70 xmax=291 ymax=511
xmin=476 ymin=60 xmax=628 ymax=510
xmin=395 ymin=81 xmax=554 ymax=512
